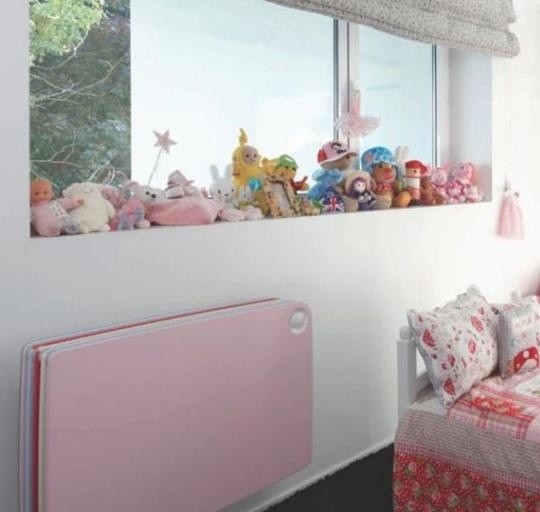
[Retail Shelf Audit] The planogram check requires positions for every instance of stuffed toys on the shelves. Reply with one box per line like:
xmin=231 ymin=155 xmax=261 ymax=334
xmin=63 ymin=164 xmax=264 ymax=234
xmin=61 ymin=128 xmax=484 ymax=234
xmin=232 ymin=127 xmax=484 ymax=218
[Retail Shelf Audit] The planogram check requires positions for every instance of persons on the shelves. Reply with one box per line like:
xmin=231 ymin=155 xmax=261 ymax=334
xmin=30 ymin=177 xmax=86 ymax=237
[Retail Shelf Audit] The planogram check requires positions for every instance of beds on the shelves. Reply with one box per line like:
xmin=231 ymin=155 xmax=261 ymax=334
xmin=397 ymin=292 xmax=540 ymax=512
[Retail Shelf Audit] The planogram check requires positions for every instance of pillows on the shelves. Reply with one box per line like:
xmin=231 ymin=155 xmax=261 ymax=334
xmin=499 ymin=294 xmax=540 ymax=379
xmin=407 ymin=282 xmax=500 ymax=412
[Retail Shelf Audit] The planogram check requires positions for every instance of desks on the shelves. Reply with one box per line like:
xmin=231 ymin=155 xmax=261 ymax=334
xmin=16 ymin=296 xmax=314 ymax=512
